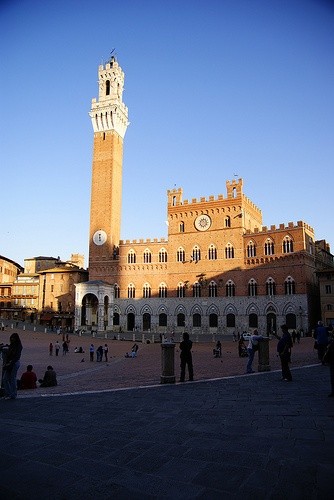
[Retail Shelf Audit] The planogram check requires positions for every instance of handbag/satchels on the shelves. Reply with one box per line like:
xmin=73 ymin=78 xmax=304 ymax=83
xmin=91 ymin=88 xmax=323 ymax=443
xmin=253 ymin=345 xmax=258 ymax=350
xmin=284 ymin=352 xmax=290 ymax=362
xmin=3 ymin=360 xmax=13 ymax=369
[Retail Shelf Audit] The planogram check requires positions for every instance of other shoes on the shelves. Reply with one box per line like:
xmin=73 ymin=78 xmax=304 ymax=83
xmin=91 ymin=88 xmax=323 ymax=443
xmin=2 ymin=394 xmax=9 ymax=399
xmin=284 ymin=379 xmax=290 ymax=381
xmin=5 ymin=395 xmax=15 ymax=400
xmin=247 ymin=370 xmax=255 ymax=373
xmin=281 ymin=376 xmax=285 ymax=379
xmin=328 ymin=392 xmax=333 ymax=397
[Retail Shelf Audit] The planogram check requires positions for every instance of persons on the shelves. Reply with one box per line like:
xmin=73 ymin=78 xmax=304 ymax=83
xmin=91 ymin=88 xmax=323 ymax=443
xmin=0 ymin=320 xmax=332 ymax=363
xmin=246 ymin=330 xmax=267 ymax=372
xmin=4 ymin=333 xmax=23 ymax=399
xmin=179 ymin=333 xmax=194 ymax=382
xmin=0 ymin=343 xmax=9 ymax=397
xmin=38 ymin=366 xmax=58 ymax=388
xmin=17 ymin=365 xmax=38 ymax=389
xmin=277 ymin=324 xmax=292 ymax=382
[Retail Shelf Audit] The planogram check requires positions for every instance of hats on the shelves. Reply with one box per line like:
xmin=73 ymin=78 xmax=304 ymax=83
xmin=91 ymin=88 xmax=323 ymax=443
xmin=281 ymin=324 xmax=288 ymax=329
